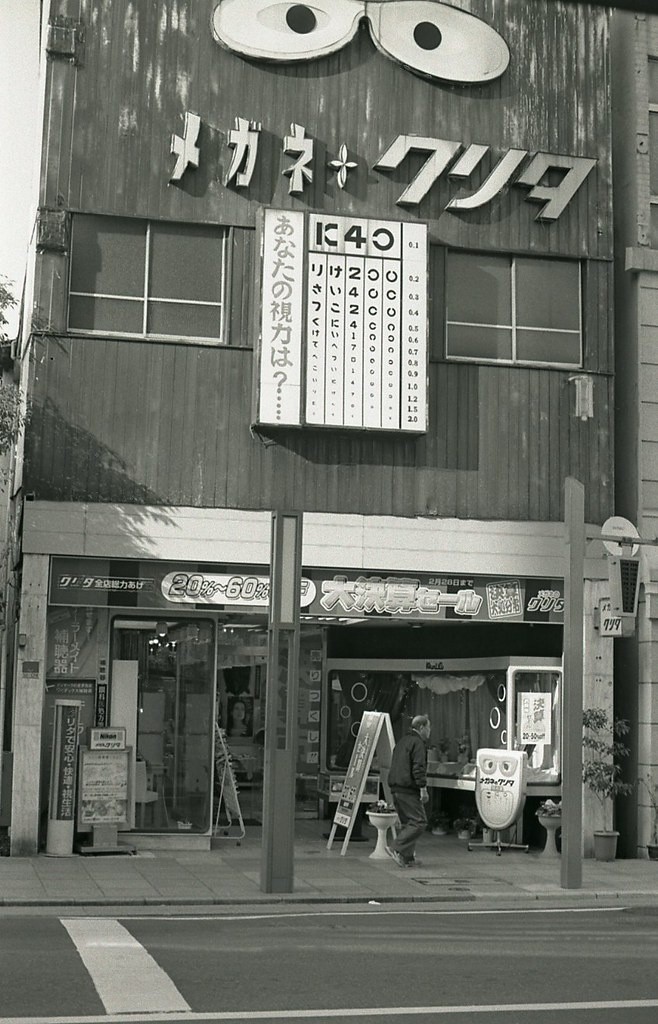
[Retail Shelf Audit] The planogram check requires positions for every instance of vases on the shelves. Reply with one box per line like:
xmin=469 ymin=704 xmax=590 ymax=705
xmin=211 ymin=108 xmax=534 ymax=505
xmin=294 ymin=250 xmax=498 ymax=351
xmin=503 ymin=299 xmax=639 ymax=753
xmin=458 ymin=753 xmax=468 ymax=764
xmin=365 ymin=812 xmax=399 ymax=858
xmin=440 ymin=752 xmax=451 ymax=763
xmin=536 ymin=814 xmax=562 ymax=856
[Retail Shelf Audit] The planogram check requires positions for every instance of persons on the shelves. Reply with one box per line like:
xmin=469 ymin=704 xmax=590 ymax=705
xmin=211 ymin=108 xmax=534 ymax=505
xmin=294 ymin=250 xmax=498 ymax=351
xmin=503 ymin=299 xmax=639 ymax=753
xmin=387 ymin=716 xmax=431 ymax=868
xmin=227 ymin=700 xmax=251 ymax=737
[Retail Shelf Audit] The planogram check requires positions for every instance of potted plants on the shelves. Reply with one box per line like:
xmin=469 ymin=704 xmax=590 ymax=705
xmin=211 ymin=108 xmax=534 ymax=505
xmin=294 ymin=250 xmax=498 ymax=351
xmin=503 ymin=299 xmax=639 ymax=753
xmin=636 ymin=773 xmax=658 ymax=862
xmin=582 ymin=706 xmax=633 ymax=862
xmin=453 ymin=817 xmax=476 ymax=839
xmin=429 ymin=814 xmax=450 ymax=836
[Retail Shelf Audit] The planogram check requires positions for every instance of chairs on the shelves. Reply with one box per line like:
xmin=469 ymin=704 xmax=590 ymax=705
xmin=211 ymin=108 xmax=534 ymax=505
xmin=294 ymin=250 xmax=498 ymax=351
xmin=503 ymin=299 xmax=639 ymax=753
xmin=136 ymin=761 xmax=158 ymax=827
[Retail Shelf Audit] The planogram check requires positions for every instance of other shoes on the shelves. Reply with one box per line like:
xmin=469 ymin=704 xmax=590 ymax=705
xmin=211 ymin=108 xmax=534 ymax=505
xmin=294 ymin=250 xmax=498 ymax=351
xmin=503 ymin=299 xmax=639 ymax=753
xmin=385 ymin=847 xmax=406 ymax=868
xmin=406 ymin=858 xmax=422 ymax=867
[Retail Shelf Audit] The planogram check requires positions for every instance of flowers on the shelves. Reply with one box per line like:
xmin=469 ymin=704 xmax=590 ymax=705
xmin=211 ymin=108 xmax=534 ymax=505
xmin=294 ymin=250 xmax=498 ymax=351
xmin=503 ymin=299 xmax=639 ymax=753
xmin=535 ymin=799 xmax=562 ymax=816
xmin=437 ymin=735 xmax=450 ymax=752
xmin=368 ymin=799 xmax=395 ymax=813
xmin=456 ymin=735 xmax=469 ymax=753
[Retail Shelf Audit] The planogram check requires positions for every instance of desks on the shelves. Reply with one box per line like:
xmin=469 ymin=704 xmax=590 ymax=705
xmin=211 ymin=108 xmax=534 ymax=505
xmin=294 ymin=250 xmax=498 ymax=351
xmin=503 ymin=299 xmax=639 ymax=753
xmin=145 ymin=765 xmax=169 ymax=827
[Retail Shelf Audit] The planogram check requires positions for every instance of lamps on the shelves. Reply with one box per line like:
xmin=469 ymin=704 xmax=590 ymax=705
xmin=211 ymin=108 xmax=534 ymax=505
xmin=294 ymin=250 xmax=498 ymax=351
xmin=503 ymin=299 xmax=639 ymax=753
xmin=569 ymin=375 xmax=595 ymax=421
